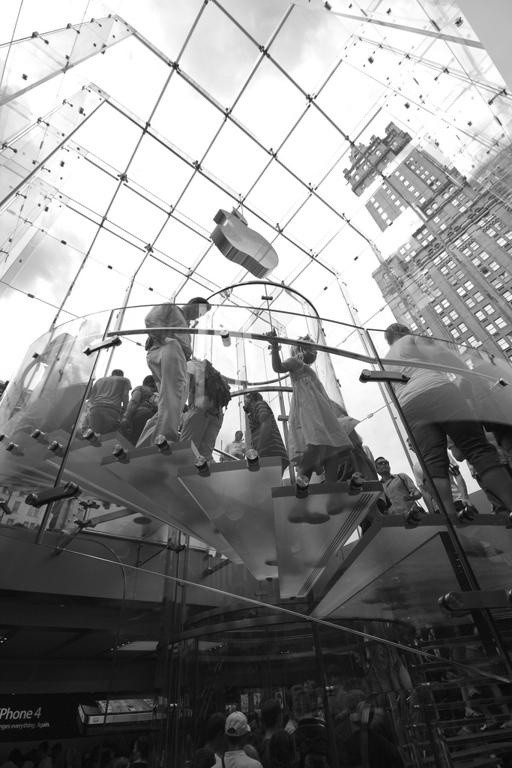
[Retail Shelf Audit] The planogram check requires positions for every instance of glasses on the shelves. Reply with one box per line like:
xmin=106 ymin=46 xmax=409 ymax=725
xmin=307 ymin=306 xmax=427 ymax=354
xmin=375 ymin=460 xmax=389 ymax=465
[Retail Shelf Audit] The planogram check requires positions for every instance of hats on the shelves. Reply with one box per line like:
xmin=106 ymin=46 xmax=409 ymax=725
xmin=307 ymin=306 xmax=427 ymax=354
xmin=226 ymin=711 xmax=251 ymax=736
xmin=262 ymin=699 xmax=281 ymax=714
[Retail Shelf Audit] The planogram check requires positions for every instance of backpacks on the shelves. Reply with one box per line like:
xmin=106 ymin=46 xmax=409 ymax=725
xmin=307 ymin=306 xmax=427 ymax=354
xmin=202 ymin=359 xmax=232 ymax=410
xmin=145 ymin=391 xmax=158 ymax=410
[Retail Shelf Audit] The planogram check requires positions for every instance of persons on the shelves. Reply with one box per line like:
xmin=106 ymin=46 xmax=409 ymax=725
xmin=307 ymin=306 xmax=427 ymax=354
xmin=2 ymin=686 xmax=402 ymax=766
xmin=145 ymin=297 xmax=421 ymax=524
xmin=364 ymin=621 xmax=511 ymax=767
xmin=41 ymin=319 xmax=158 ymax=445
xmin=382 ymin=322 xmax=511 ymax=513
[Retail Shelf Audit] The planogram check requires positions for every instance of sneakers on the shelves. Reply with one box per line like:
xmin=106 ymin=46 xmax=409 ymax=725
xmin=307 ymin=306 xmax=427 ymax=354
xmin=288 ymin=508 xmax=330 ymax=524
xmin=327 ymin=496 xmax=369 ymax=515
